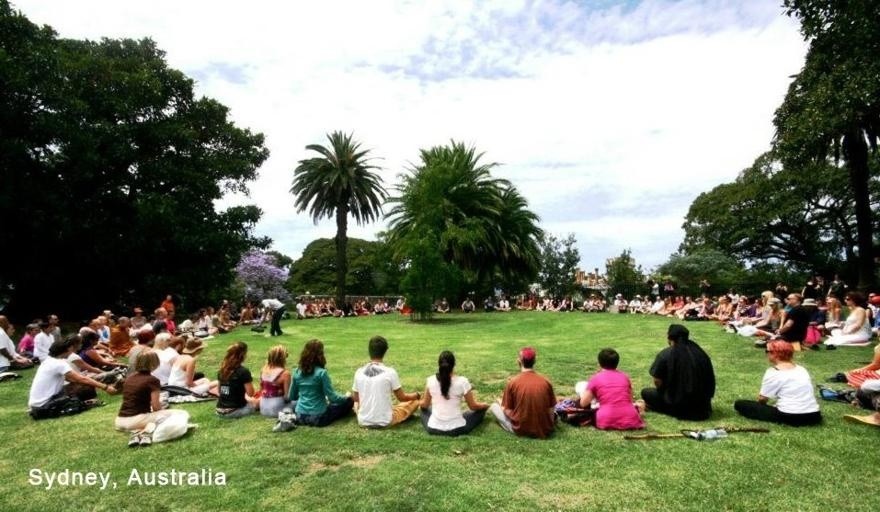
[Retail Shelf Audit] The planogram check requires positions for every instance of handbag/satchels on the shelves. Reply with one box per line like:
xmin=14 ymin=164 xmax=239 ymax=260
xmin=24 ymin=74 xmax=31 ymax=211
xmin=558 ymin=398 xmax=593 ymax=427
xmin=272 ymin=401 xmax=298 ymax=432
xmin=29 ymin=395 xmax=87 ymax=421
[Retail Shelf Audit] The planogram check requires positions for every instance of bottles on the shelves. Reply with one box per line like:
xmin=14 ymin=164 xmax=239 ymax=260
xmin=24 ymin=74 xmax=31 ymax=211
xmin=700 ymin=428 xmax=729 ymax=439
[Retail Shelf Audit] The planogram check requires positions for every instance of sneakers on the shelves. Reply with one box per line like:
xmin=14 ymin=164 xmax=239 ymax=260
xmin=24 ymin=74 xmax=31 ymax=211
xmin=141 ymin=436 xmax=152 ymax=444
xmin=128 ymin=435 xmax=139 ymax=446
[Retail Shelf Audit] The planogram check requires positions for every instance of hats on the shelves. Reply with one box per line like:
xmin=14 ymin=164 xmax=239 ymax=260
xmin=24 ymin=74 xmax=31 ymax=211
xmin=668 ymin=324 xmax=689 ymax=338
xmin=183 ymin=337 xmax=208 ymax=354
xmin=517 ymin=346 xmax=535 ymax=360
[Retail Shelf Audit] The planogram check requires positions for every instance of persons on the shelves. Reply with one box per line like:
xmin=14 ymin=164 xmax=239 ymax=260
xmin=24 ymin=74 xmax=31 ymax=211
xmin=0 ymin=278 xmax=880 ymax=447
